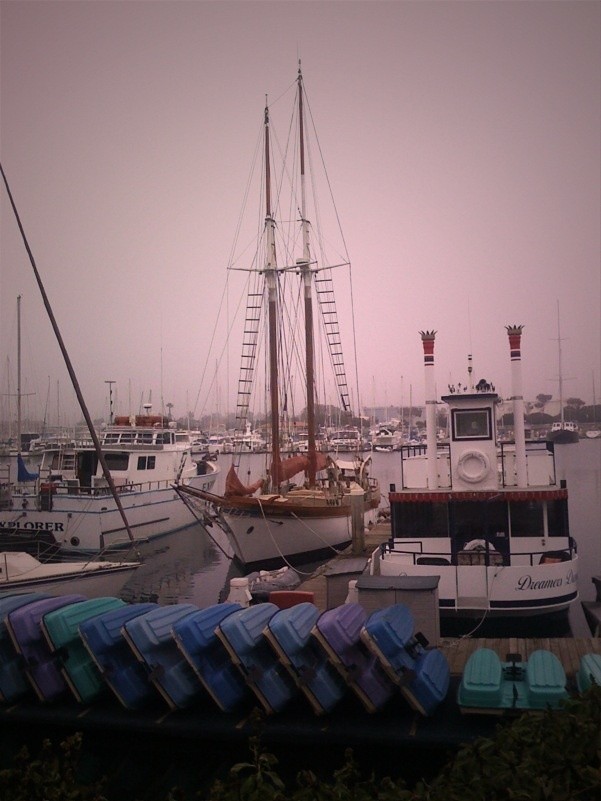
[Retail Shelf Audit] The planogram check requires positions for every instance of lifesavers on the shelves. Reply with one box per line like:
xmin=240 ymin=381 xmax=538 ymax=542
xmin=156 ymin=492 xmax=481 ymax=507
xmin=462 ymin=538 xmax=496 ymax=566
xmin=457 ymin=449 xmax=491 ymax=483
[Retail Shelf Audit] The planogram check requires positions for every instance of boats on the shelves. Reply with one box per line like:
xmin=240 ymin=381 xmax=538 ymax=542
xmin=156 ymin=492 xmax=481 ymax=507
xmin=2 ymin=345 xmax=601 ymax=457
xmin=0 ymin=589 xmax=601 ymax=720
xmin=0 ymin=295 xmax=221 ymax=554
xmin=355 ymin=324 xmax=582 ymax=622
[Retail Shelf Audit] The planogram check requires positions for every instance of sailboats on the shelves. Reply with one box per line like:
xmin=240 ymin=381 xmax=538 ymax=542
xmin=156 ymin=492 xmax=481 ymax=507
xmin=168 ymin=58 xmax=382 ymax=567
